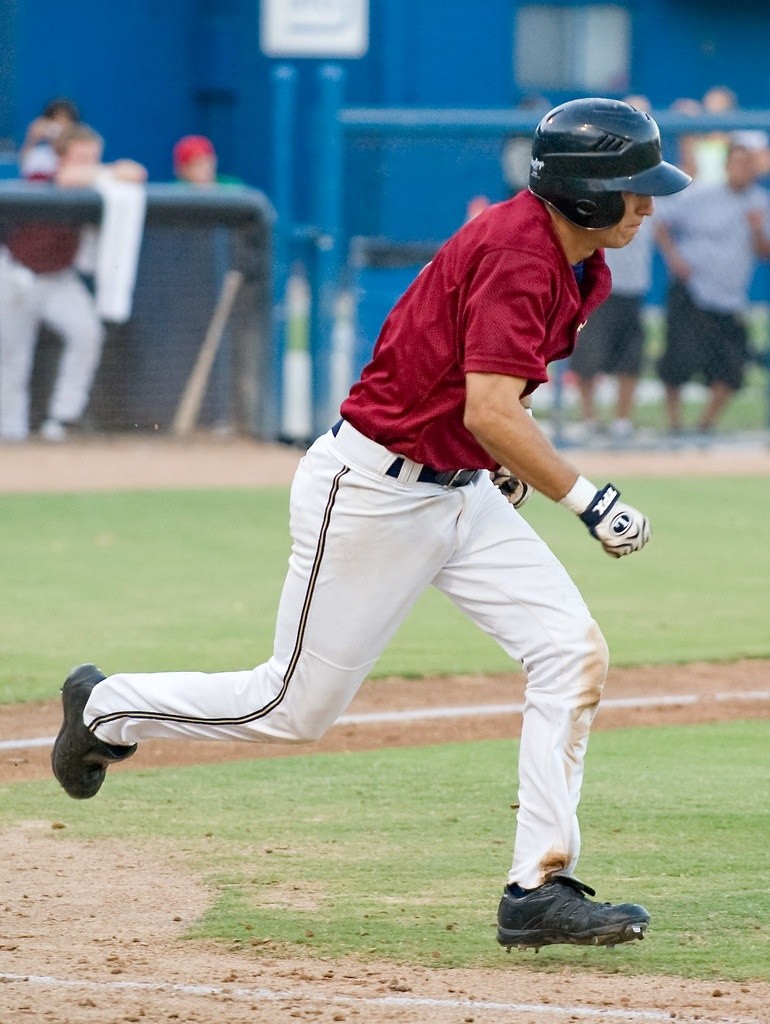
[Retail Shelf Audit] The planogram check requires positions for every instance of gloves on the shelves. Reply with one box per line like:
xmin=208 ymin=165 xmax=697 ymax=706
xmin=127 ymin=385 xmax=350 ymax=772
xmin=556 ymin=474 xmax=654 ymax=558
xmin=494 ymin=466 xmax=533 ymax=510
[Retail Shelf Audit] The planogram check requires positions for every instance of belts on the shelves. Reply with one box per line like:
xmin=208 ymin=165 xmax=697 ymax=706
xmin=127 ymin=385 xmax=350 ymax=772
xmin=329 ymin=418 xmax=483 ymax=490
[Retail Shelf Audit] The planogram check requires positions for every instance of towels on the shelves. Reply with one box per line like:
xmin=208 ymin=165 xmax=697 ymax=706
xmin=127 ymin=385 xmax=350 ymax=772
xmin=67 ymin=172 xmax=149 ymax=323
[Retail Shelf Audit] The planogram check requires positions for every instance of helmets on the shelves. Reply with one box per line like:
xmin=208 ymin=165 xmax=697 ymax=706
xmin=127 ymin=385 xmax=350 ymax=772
xmin=525 ymin=97 xmax=694 ymax=231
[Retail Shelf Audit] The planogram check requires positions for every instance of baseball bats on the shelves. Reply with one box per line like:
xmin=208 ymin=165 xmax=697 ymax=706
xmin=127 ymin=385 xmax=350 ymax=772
xmin=175 ymin=270 xmax=244 ymax=434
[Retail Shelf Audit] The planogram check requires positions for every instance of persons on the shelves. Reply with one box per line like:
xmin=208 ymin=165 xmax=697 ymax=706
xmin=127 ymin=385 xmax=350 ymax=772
xmin=1 ymin=84 xmax=770 ymax=440
xmin=52 ymin=100 xmax=693 ymax=945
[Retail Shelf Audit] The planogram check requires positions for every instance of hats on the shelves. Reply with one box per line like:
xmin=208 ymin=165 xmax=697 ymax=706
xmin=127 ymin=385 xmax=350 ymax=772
xmin=171 ymin=133 xmax=217 ymax=169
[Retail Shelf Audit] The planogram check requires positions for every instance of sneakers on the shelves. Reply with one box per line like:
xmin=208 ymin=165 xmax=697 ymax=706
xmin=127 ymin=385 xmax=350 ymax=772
xmin=496 ymin=873 xmax=653 ymax=954
xmin=50 ymin=663 xmax=138 ymax=800
